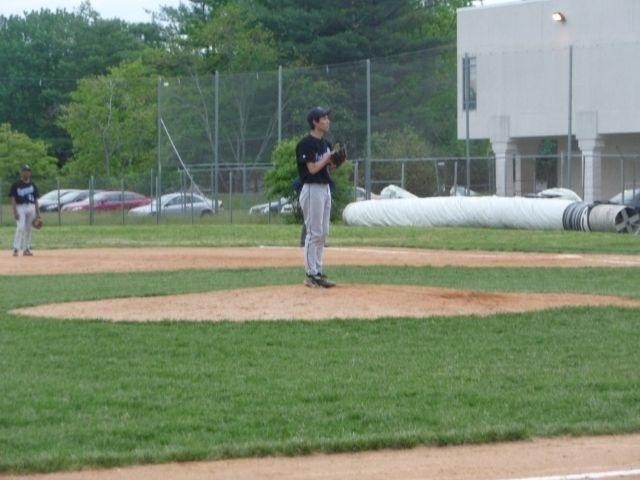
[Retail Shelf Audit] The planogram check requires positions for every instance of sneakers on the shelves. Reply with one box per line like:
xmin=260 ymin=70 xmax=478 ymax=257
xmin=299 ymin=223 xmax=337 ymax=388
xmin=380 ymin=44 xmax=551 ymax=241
xmin=304 ymin=274 xmax=335 ymax=288
xmin=13 ymin=250 xmax=34 ymax=257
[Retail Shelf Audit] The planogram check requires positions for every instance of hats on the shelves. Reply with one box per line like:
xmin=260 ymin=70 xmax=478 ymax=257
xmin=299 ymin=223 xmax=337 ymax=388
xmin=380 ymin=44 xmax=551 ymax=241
xmin=21 ymin=164 xmax=31 ymax=170
xmin=307 ymin=106 xmax=330 ymax=122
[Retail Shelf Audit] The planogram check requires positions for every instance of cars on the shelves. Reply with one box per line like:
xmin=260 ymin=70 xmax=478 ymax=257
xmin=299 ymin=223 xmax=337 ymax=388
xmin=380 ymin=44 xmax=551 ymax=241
xmin=37 ymin=188 xmax=223 ymax=222
xmin=250 ymin=185 xmax=384 ymax=220
xmin=609 ymin=188 xmax=640 ymax=207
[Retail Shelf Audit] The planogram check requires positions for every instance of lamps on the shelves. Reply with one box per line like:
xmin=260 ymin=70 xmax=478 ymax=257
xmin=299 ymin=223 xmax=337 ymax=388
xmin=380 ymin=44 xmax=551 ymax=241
xmin=550 ymin=11 xmax=566 ymax=24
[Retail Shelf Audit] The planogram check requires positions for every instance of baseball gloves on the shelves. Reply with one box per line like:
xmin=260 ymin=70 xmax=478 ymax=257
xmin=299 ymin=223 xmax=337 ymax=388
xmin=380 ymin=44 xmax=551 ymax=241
xmin=331 ymin=142 xmax=346 ymax=166
xmin=33 ymin=217 xmax=43 ymax=229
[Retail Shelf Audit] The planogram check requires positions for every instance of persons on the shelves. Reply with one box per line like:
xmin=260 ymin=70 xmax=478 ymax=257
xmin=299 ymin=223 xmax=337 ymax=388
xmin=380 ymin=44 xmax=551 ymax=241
xmin=291 ymin=174 xmax=336 ymax=247
xmin=295 ymin=106 xmax=345 ymax=290
xmin=7 ymin=164 xmax=42 ymax=258
